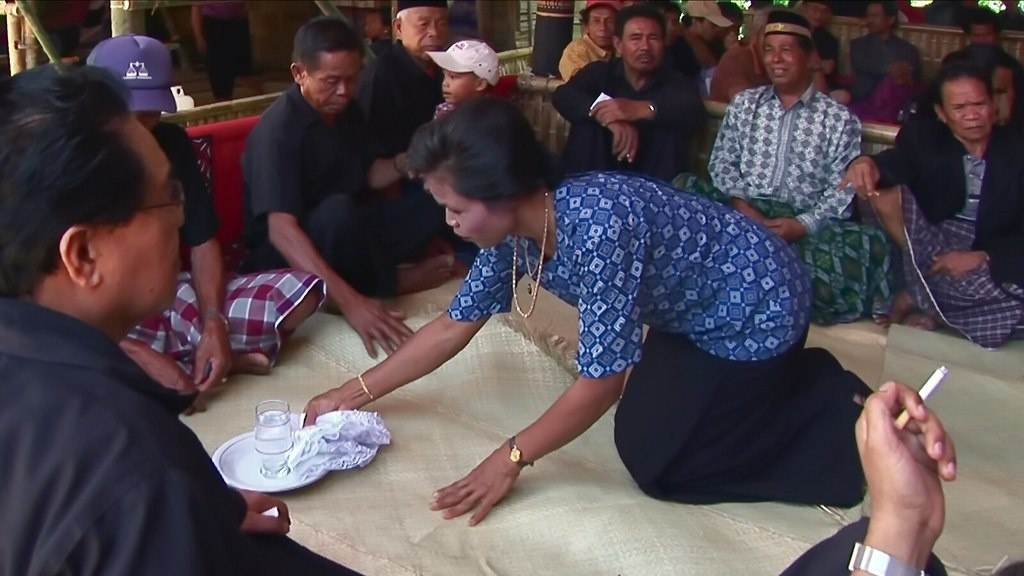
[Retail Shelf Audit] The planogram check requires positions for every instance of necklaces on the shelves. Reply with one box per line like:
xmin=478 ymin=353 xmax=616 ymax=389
xmin=510 ymin=187 xmax=552 ymax=319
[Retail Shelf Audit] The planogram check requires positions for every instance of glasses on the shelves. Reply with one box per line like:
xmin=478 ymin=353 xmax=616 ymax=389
xmin=137 ymin=180 xmax=184 ymax=215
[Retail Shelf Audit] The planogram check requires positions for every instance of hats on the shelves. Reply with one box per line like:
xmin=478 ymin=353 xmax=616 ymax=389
xmin=764 ymin=10 xmax=811 ymax=42
xmin=85 ymin=34 xmax=177 ymax=115
xmin=424 ymin=40 xmax=500 ymax=87
xmin=685 ymin=0 xmax=733 ymax=28
xmin=395 ymin=0 xmax=448 ymax=14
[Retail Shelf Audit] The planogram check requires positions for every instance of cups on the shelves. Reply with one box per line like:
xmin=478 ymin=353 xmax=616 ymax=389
xmin=254 ymin=399 xmax=294 ymax=480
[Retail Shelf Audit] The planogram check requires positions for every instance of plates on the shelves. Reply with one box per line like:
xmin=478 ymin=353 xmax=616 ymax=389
xmin=211 ymin=430 xmax=329 ymax=494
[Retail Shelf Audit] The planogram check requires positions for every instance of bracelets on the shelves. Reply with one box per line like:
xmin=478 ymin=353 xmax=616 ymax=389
xmin=357 ymin=372 xmax=376 ymax=401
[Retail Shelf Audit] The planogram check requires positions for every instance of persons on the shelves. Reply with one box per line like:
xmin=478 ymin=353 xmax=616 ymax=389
xmin=0 ymin=61 xmax=308 ymax=576
xmin=49 ymin=31 xmax=329 ymax=412
xmin=769 ymin=370 xmax=1024 ymax=576
xmin=303 ymin=98 xmax=879 ymax=528
xmin=191 ymin=1 xmax=1024 ymax=347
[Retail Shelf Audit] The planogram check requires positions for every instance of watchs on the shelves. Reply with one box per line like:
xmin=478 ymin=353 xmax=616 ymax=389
xmin=507 ymin=435 xmax=533 ymax=469
xmin=846 ymin=540 xmax=926 ymax=576
